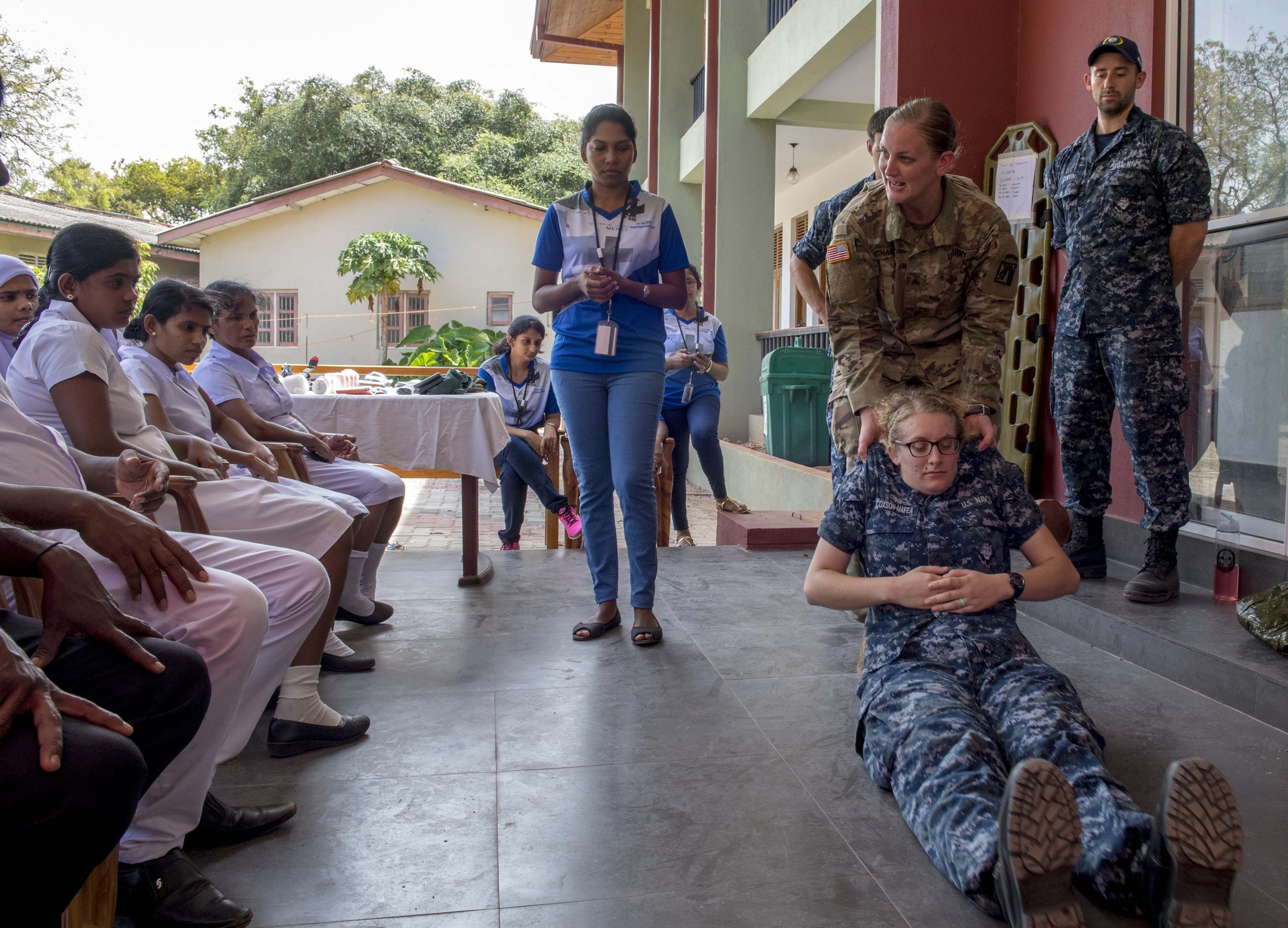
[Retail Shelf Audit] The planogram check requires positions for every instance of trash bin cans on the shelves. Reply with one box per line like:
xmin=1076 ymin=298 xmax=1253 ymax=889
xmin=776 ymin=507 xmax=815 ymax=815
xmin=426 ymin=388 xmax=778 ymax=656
xmin=758 ymin=337 xmax=835 ymax=467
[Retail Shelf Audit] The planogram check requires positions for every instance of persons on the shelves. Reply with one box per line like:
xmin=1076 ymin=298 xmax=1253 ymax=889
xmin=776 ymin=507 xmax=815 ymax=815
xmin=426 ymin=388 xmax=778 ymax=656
xmin=9 ymin=222 xmax=372 ymax=761
xmin=478 ymin=314 xmax=581 ymax=551
xmin=117 ymin=276 xmax=377 ymax=673
xmin=819 ymin=97 xmax=1022 ymax=483
xmin=787 ymin=107 xmax=903 ymax=503
xmin=1 ymin=373 xmax=334 ymax=926
xmin=1042 ymin=34 xmax=1210 ymax=604
xmin=0 ymin=252 xmax=40 ymax=378
xmin=804 ymin=386 xmax=1243 ymax=928
xmin=531 ymin=103 xmax=692 ymax=650
xmin=0 ymin=524 xmax=213 ymax=928
xmin=189 ymin=279 xmax=408 ymax=625
xmin=663 ymin=262 xmax=750 ymax=549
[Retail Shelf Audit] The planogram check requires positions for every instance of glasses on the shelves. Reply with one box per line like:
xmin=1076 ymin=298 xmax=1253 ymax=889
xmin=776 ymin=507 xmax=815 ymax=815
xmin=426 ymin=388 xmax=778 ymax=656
xmin=686 ymin=278 xmax=697 ymax=287
xmin=891 ymin=436 xmax=963 ymax=458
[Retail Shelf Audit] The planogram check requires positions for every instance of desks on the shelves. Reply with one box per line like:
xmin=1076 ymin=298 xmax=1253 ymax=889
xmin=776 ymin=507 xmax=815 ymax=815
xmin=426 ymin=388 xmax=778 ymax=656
xmin=289 ymin=390 xmax=512 ymax=587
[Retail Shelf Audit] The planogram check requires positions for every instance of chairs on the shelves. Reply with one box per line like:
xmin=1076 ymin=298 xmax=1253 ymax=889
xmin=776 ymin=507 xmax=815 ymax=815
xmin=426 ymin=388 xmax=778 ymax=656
xmin=560 ymin=435 xmax=676 ymax=550
xmin=1 ymin=432 xmax=361 ymax=928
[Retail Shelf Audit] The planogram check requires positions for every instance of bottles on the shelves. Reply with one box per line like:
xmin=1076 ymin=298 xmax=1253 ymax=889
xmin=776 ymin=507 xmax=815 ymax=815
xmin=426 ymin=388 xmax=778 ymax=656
xmin=1213 ymin=512 xmax=1240 ymax=601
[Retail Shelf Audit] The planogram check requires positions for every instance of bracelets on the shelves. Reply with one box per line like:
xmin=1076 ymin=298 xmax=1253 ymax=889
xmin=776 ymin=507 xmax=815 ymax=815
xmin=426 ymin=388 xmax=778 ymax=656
xmin=28 ymin=540 xmax=63 ymax=578
xmin=963 ymin=405 xmax=992 ymax=422
xmin=320 ymin=435 xmax=328 ymax=441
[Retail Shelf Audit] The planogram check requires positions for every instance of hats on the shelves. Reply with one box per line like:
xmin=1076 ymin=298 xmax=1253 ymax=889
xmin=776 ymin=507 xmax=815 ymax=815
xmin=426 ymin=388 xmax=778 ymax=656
xmin=1087 ymin=34 xmax=1142 ymax=72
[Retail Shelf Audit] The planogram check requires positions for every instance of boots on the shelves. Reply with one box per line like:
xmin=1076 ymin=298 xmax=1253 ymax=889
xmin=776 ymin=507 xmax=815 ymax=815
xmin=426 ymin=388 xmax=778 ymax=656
xmin=1024 ymin=510 xmax=1180 ymax=602
xmin=993 ymin=754 xmax=1245 ymax=928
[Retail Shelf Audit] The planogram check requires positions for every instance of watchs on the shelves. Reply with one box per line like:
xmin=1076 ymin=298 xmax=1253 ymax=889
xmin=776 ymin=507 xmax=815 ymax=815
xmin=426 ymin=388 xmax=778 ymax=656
xmin=544 ymin=423 xmax=556 ymax=430
xmin=1007 ymin=572 xmax=1025 ymax=600
xmin=637 ymin=282 xmax=651 ymax=302
xmin=705 ymin=360 xmax=713 ymax=373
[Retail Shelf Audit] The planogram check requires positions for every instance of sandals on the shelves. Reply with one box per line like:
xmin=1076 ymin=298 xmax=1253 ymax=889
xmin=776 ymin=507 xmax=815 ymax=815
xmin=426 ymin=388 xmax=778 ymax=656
xmin=572 ymin=605 xmax=663 ymax=645
xmin=676 ymin=498 xmax=751 ymax=547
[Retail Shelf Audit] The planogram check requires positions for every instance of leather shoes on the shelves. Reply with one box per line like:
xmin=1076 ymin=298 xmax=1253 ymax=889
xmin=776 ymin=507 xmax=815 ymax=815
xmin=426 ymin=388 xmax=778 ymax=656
xmin=118 ymin=790 xmax=297 ymax=928
xmin=335 ymin=593 xmax=393 ymax=626
xmin=263 ymin=712 xmax=370 ymax=758
xmin=321 ymin=636 xmax=377 ymax=672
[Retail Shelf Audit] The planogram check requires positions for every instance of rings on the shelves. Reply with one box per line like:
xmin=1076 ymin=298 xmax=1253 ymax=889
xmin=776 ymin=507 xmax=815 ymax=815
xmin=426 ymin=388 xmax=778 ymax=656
xmin=702 ymin=367 xmax=706 ymax=370
xmin=959 ymin=598 xmax=966 ymax=607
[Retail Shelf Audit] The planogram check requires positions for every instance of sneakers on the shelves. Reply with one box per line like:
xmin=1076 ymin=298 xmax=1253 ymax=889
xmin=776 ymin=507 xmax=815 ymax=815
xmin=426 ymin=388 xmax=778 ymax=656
xmin=500 ymin=502 xmax=584 ymax=551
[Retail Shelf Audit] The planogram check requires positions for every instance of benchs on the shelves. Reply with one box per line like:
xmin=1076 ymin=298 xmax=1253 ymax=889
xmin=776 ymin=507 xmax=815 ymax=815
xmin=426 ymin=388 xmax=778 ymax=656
xmin=180 ymin=363 xmax=565 ymax=549
